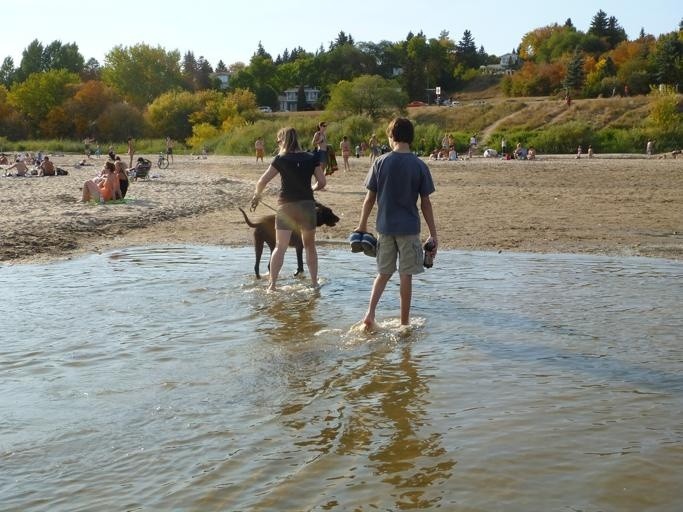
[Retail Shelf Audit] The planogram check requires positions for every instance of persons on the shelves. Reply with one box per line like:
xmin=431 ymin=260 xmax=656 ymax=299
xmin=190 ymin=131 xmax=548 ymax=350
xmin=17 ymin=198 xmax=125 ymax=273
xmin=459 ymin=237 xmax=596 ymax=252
xmin=250 ymin=126 xmax=326 ymax=293
xmin=565 ymin=92 xmax=570 ymax=108
xmin=646 ymin=139 xmax=652 ymax=159
xmin=355 ymin=130 xmax=536 ymax=163
xmin=166 ymin=136 xmax=175 ymax=165
xmin=0 ymin=134 xmax=148 ymax=206
xmin=352 ymin=116 xmax=438 ymax=332
xmin=255 ymin=137 xmax=263 ymax=164
xmin=577 ymin=145 xmax=581 ymax=158
xmin=340 ymin=136 xmax=352 ymax=172
xmin=625 ymin=84 xmax=628 ymax=97
xmin=588 ymin=145 xmax=593 ymax=159
xmin=312 ymin=121 xmax=331 ymax=192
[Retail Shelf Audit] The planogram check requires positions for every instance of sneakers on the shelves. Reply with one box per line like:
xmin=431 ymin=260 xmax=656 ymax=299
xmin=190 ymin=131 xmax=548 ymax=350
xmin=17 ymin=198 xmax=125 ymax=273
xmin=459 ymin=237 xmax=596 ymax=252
xmin=350 ymin=230 xmax=363 ymax=253
xmin=360 ymin=231 xmax=377 ymax=257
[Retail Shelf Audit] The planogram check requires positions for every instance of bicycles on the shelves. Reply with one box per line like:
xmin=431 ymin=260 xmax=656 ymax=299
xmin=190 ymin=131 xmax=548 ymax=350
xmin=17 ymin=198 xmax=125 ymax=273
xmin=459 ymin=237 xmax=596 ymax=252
xmin=156 ymin=152 xmax=169 ymax=170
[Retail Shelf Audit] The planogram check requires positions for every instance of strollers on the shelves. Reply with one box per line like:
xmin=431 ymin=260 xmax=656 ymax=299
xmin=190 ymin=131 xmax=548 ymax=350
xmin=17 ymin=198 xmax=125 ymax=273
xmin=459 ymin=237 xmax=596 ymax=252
xmin=127 ymin=159 xmax=151 ymax=181
xmin=377 ymin=145 xmax=390 ymax=155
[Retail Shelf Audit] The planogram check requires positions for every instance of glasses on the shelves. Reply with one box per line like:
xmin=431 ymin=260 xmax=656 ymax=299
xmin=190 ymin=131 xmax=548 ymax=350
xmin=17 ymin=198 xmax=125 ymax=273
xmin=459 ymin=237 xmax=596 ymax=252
xmin=322 ymin=124 xmax=327 ymax=127
xmin=276 ymin=139 xmax=283 ymax=144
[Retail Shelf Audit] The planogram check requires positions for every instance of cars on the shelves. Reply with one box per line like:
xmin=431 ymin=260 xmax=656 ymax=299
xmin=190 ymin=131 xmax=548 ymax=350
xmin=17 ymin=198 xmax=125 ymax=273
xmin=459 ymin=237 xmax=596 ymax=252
xmin=256 ymin=106 xmax=271 ymax=113
xmin=405 ymin=100 xmax=429 ymax=107
xmin=434 ymin=98 xmax=461 ymax=106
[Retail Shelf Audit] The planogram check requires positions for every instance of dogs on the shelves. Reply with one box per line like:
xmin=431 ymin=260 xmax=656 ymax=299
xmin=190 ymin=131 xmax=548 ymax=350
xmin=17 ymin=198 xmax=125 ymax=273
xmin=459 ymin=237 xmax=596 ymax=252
xmin=238 ymin=202 xmax=340 ymax=279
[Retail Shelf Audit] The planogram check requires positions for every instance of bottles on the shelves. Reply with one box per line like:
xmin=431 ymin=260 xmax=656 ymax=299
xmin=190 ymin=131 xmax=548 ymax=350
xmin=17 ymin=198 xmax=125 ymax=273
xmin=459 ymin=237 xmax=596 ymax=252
xmin=423 ymin=236 xmax=434 ymax=269
xmin=98 ymin=191 xmax=104 ymax=204
